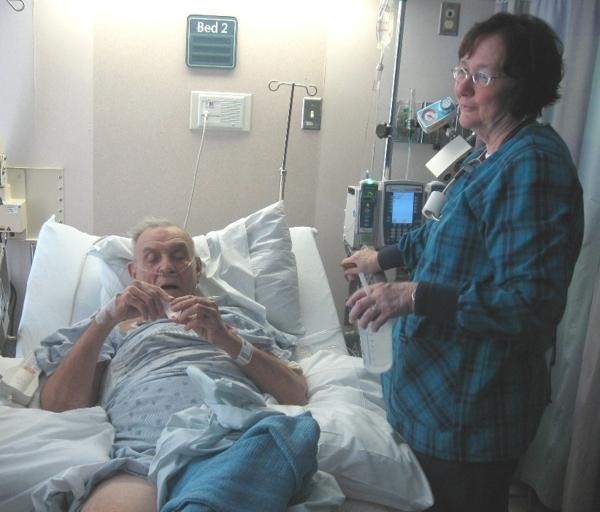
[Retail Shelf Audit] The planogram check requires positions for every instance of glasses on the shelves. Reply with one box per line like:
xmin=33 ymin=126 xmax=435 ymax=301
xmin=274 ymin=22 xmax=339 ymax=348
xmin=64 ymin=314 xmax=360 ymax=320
xmin=450 ymin=66 xmax=510 ymax=88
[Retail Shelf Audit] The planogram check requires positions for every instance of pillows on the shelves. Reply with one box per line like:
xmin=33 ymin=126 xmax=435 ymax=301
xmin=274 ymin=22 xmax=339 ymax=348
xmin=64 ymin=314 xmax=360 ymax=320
xmin=16 ymin=200 xmax=306 ymax=358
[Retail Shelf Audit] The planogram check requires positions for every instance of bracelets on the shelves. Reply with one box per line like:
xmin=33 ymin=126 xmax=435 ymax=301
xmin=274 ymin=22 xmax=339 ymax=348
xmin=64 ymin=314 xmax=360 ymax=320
xmin=234 ymin=335 xmax=254 ymax=367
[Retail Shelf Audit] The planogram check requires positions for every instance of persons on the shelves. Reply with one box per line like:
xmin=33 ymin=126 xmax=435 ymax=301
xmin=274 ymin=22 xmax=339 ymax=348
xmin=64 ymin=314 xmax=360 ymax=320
xmin=38 ymin=219 xmax=320 ymax=512
xmin=342 ymin=10 xmax=585 ymax=510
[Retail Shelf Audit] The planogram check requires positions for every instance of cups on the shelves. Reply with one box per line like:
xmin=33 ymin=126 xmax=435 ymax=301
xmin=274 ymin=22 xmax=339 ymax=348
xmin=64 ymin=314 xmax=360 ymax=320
xmin=358 ymin=320 xmax=394 ymax=374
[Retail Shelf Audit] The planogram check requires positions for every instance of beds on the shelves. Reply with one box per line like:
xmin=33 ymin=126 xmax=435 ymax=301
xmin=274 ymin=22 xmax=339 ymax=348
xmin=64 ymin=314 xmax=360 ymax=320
xmin=1 ymin=225 xmax=434 ymax=511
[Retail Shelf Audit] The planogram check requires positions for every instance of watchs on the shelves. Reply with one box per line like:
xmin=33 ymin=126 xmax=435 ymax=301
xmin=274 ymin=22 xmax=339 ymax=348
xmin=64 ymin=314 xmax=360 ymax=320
xmin=408 ymin=288 xmax=417 ymax=317
